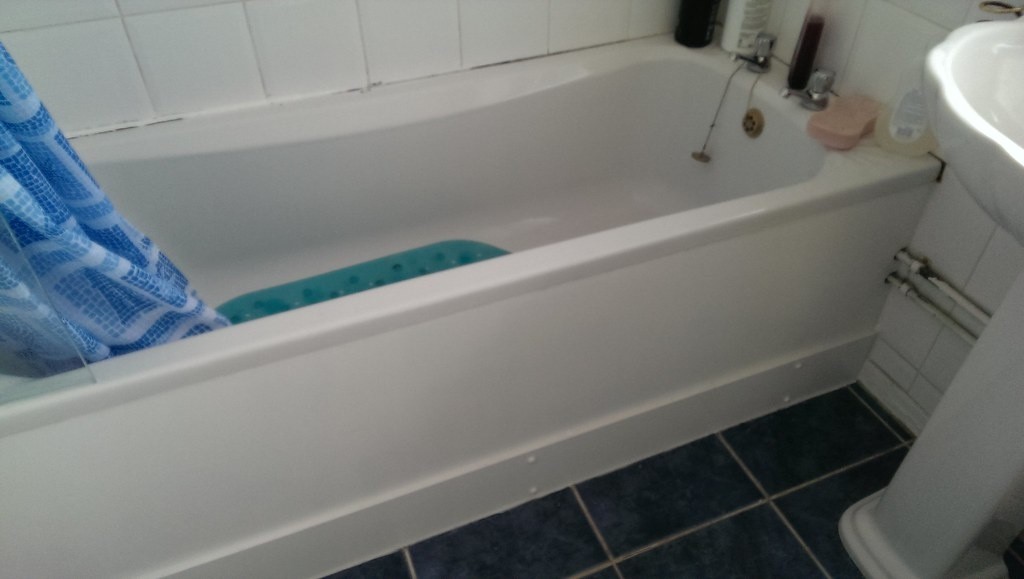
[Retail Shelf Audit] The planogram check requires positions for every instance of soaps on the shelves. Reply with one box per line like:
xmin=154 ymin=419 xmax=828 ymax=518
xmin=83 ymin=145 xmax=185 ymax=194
xmin=806 ymin=93 xmax=883 ymax=152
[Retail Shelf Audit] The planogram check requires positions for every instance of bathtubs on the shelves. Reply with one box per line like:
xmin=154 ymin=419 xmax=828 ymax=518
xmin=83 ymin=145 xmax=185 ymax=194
xmin=1 ymin=30 xmax=946 ymax=579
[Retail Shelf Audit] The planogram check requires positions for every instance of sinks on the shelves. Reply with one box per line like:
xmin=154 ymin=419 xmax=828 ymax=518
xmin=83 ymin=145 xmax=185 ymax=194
xmin=920 ymin=19 xmax=1022 ymax=248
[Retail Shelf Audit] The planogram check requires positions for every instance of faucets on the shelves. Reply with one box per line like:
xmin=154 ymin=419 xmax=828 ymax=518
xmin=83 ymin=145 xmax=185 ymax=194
xmin=726 ymin=30 xmax=836 ymax=112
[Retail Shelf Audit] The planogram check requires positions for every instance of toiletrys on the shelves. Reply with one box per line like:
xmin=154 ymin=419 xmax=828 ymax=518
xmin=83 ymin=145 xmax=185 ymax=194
xmin=873 ymin=24 xmax=952 ymax=160
xmin=673 ymin=0 xmax=774 ymax=56
